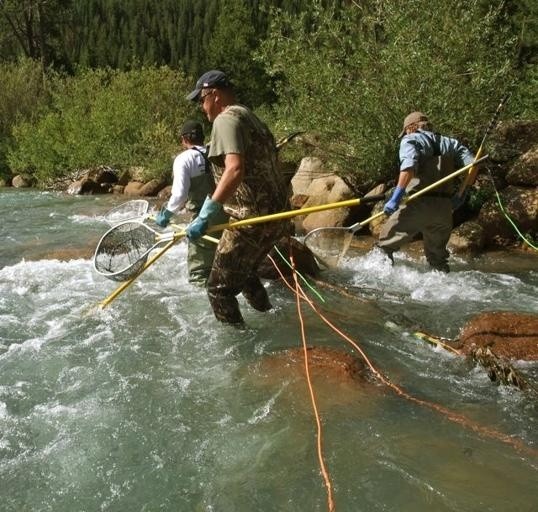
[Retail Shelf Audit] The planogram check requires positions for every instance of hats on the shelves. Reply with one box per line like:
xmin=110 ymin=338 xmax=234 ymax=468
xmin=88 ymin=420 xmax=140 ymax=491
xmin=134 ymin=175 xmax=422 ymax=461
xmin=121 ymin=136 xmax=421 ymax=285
xmin=180 ymin=121 xmax=204 ymax=136
xmin=185 ymin=69 xmax=234 ymax=100
xmin=397 ymin=110 xmax=429 ymax=139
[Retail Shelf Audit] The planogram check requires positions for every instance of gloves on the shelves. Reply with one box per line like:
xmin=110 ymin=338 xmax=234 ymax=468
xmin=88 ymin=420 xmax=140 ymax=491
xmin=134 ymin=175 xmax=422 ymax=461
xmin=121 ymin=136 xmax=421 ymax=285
xmin=452 ymin=184 xmax=473 ymax=211
xmin=185 ymin=193 xmax=224 ymax=244
xmin=155 ymin=206 xmax=175 ymax=225
xmin=383 ymin=186 xmax=404 ymax=216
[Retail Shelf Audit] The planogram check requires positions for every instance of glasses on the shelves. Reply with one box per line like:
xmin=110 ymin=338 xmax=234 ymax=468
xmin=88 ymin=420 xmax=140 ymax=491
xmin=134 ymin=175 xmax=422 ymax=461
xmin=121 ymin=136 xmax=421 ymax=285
xmin=198 ymin=92 xmax=213 ymax=103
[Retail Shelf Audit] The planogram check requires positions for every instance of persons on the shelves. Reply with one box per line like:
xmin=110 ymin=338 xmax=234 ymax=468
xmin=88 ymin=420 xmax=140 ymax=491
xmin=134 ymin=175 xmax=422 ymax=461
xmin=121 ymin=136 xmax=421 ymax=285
xmin=373 ymin=110 xmax=479 ymax=275
xmin=153 ymin=119 xmax=231 ymax=288
xmin=183 ymin=70 xmax=291 ymax=328
